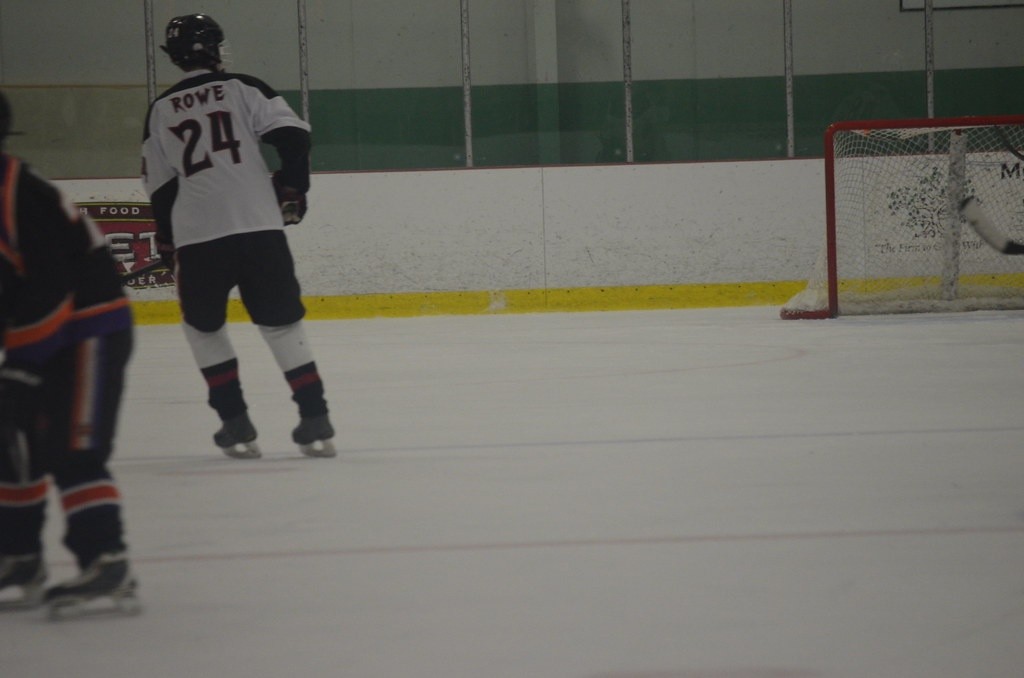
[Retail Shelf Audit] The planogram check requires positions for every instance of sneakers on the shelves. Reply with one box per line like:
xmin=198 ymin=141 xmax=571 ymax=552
xmin=213 ymin=409 xmax=260 ymax=458
xmin=292 ymin=405 xmax=335 ymax=456
xmin=45 ymin=550 xmax=140 ymax=619
xmin=0 ymin=554 xmax=44 ymax=610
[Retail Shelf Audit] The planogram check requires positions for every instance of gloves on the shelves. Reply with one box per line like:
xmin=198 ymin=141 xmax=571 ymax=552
xmin=272 ymin=172 xmax=305 ymax=225
xmin=153 ymin=229 xmax=178 ymax=273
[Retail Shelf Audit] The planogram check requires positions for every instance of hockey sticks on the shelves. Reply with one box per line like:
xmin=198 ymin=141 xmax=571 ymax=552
xmin=957 ymin=195 xmax=1024 ymax=256
xmin=118 ymin=196 xmax=306 ymax=284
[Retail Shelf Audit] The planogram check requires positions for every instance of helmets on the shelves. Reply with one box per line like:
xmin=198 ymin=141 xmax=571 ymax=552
xmin=167 ymin=15 xmax=230 ymax=70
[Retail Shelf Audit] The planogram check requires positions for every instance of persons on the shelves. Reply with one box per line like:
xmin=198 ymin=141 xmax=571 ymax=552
xmin=141 ymin=14 xmax=338 ymax=457
xmin=0 ymin=96 xmax=141 ymax=619
